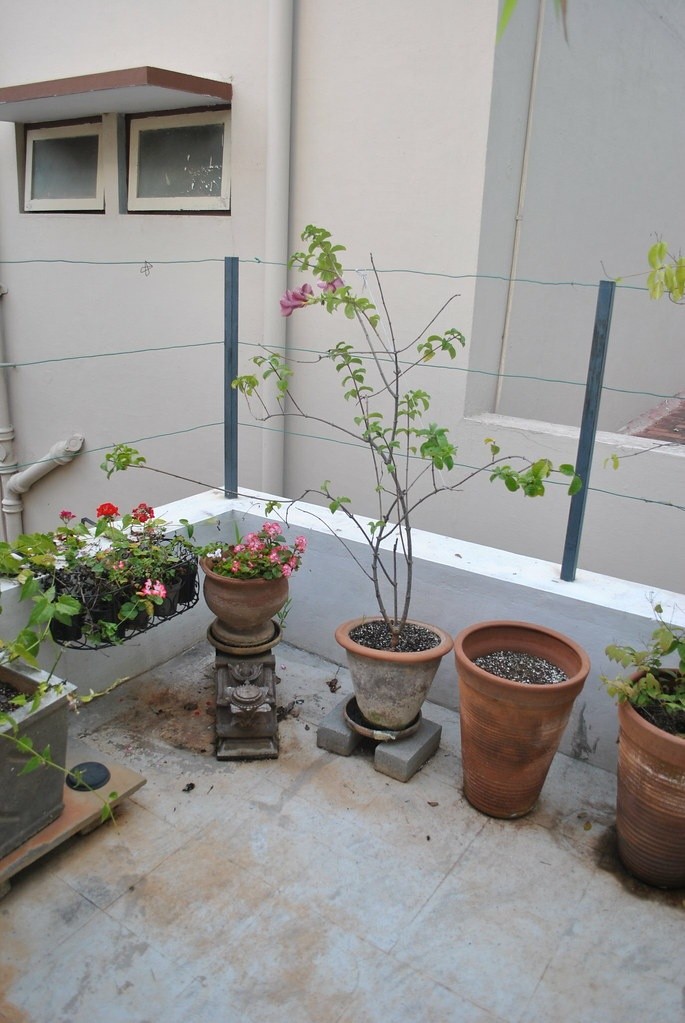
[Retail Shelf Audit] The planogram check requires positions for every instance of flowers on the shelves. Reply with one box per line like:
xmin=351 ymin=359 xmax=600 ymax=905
xmin=227 ymin=222 xmax=584 ymax=648
xmin=213 ymin=518 xmax=308 ymax=582
xmin=51 ymin=501 xmax=221 ymax=649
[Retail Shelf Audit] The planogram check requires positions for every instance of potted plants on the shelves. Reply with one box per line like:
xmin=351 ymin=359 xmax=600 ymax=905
xmin=600 ymin=589 xmax=685 ymax=891
xmin=0 ymin=529 xmax=139 ymax=862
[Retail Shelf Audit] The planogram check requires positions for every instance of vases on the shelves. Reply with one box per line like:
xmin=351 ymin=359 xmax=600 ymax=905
xmin=198 ymin=544 xmax=290 ymax=657
xmin=334 ymin=617 xmax=454 ymax=743
xmin=451 ymin=620 xmax=591 ymax=820
xmin=37 ymin=536 xmax=201 ymax=651
